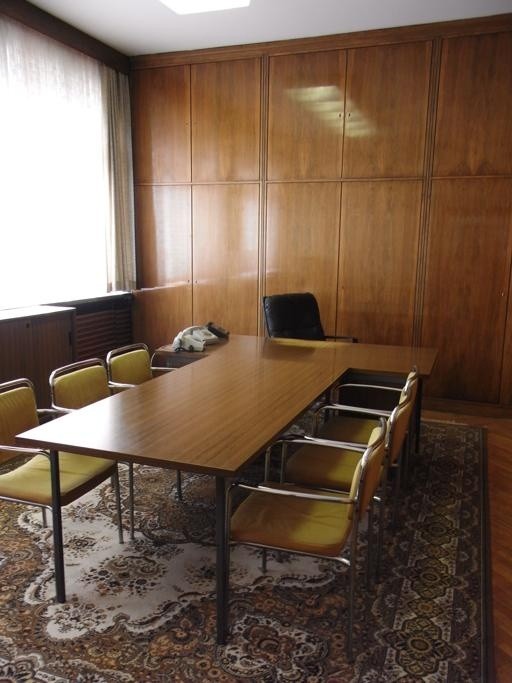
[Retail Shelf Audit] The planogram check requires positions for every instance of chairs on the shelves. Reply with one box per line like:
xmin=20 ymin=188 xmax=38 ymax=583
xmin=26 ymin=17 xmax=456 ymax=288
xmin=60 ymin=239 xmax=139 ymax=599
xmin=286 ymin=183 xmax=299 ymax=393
xmin=260 ymin=292 xmax=356 ymax=342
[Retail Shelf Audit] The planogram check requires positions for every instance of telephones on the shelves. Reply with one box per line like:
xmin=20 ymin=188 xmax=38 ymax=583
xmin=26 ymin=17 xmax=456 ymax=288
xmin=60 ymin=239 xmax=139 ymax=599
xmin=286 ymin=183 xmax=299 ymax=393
xmin=172 ymin=326 xmax=218 ymax=353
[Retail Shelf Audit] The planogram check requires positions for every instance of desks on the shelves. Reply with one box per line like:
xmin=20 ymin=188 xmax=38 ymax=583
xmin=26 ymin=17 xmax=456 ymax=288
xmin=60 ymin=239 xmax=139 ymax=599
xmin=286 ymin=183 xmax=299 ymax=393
xmin=14 ymin=326 xmax=441 ymax=642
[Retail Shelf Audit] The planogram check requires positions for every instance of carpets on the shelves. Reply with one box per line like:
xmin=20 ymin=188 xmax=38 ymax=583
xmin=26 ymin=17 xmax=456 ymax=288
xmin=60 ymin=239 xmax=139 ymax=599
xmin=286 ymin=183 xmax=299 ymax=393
xmin=2 ymin=406 xmax=498 ymax=681
